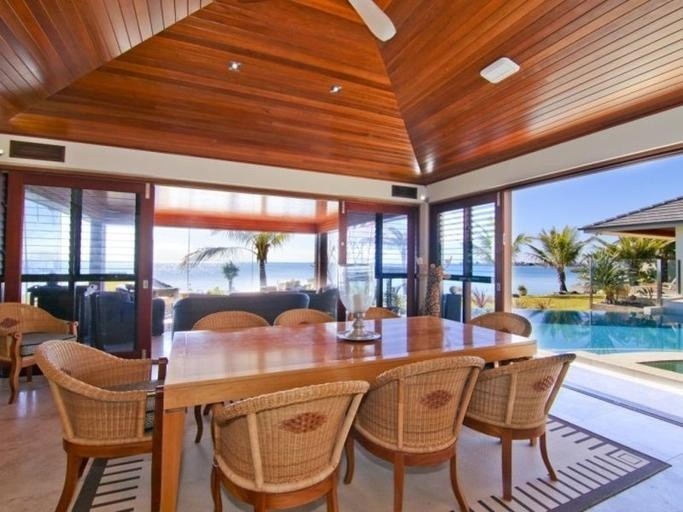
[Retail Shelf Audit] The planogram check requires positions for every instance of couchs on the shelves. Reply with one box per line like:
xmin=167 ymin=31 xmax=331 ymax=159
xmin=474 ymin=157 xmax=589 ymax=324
xmin=29 ymin=285 xmax=98 ymax=336
xmin=94 ymin=295 xmax=164 ymax=344
xmin=285 ymin=285 xmax=338 ymax=318
xmin=172 ymin=292 xmax=310 ymax=343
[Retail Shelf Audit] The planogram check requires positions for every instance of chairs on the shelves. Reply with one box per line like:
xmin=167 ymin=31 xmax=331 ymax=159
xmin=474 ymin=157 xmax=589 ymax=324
xmin=190 ymin=311 xmax=269 ymax=443
xmin=274 ymin=309 xmax=333 ymax=326
xmin=34 ymin=340 xmax=168 ymax=512
xmin=463 ymin=353 xmax=577 ymax=501
xmin=211 ymin=380 xmax=369 ymax=511
xmin=348 ymin=307 xmax=400 ymax=321
xmin=466 ymin=313 xmax=532 ymax=338
xmin=344 ymin=355 xmax=485 ymax=511
xmin=0 ymin=299 xmax=78 ymax=405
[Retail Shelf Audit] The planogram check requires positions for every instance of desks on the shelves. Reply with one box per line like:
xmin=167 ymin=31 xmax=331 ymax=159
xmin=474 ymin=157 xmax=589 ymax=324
xmin=149 ymin=314 xmax=536 ymax=511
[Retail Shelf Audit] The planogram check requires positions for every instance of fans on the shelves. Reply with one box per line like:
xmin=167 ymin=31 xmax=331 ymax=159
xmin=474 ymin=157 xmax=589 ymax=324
xmin=347 ymin=0 xmax=398 ymax=42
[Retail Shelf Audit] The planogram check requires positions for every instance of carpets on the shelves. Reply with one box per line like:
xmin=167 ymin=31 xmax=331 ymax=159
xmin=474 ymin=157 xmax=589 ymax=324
xmin=64 ymin=405 xmax=673 ymax=510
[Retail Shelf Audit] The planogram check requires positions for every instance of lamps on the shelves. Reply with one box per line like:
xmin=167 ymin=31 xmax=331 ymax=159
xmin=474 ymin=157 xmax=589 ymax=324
xmin=335 ymin=264 xmax=383 ymax=343
